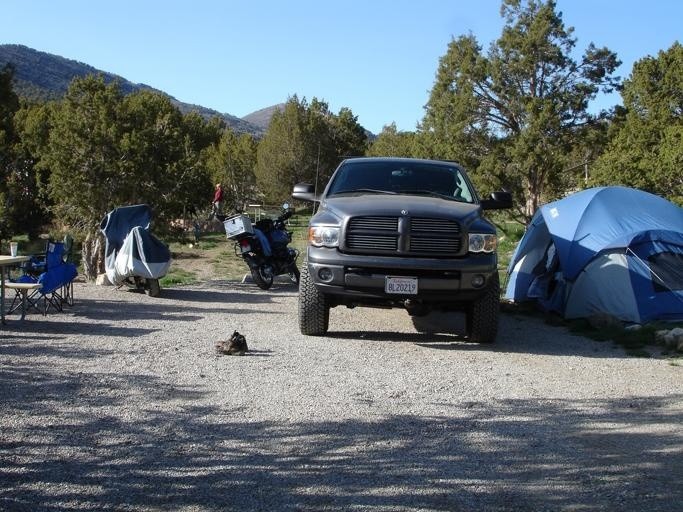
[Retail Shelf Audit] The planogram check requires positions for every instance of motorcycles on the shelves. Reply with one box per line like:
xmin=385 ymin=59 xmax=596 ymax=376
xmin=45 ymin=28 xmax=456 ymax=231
xmin=96 ymin=204 xmax=171 ymax=296
xmin=222 ymin=203 xmax=300 ymax=290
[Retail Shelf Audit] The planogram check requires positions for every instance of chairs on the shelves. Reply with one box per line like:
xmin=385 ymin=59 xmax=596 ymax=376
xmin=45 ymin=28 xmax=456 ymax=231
xmin=6 ymin=240 xmax=78 ymax=321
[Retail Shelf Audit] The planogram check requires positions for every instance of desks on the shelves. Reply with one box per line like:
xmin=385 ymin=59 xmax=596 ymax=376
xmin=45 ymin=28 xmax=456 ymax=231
xmin=0 ymin=254 xmax=30 ymax=325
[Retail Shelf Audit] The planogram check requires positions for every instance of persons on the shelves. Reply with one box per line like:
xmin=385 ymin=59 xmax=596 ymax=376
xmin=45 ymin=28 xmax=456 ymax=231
xmin=210 ymin=183 xmax=223 ymax=218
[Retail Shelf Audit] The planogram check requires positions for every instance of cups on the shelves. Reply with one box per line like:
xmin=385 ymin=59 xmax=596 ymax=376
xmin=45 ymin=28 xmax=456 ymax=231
xmin=10 ymin=241 xmax=18 ymax=257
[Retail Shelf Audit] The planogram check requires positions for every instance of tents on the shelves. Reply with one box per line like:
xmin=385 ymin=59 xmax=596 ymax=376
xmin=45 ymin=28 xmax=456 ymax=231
xmin=499 ymin=184 xmax=682 ymax=329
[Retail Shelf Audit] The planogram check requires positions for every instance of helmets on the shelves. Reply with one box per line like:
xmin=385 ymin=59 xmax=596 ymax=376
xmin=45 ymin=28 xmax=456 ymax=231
xmin=271 ymin=229 xmax=289 ymax=247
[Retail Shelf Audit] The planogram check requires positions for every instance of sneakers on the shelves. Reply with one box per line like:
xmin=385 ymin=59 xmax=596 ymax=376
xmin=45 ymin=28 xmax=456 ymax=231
xmin=214 ymin=330 xmax=248 ymax=356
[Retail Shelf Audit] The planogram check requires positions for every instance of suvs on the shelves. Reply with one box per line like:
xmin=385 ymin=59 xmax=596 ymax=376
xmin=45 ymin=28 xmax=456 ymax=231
xmin=292 ymin=155 xmax=514 ymax=343
